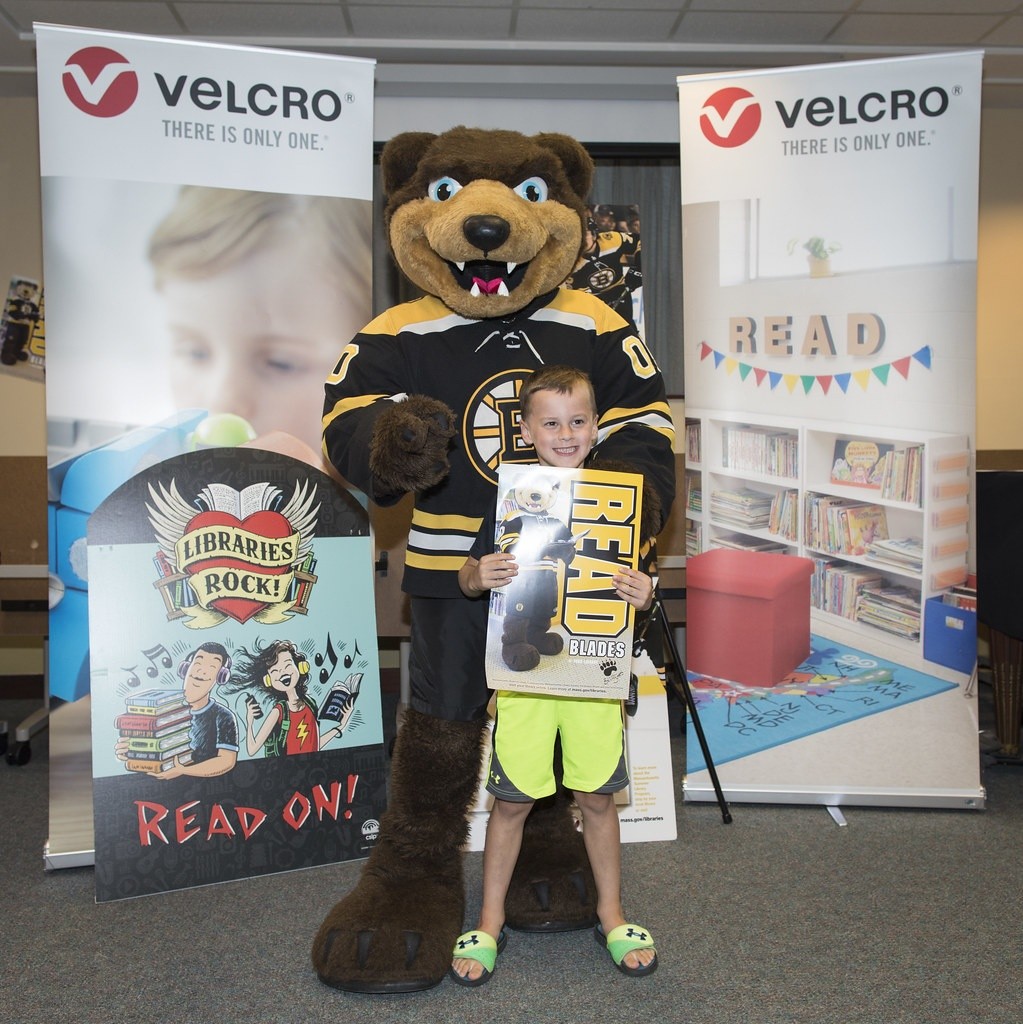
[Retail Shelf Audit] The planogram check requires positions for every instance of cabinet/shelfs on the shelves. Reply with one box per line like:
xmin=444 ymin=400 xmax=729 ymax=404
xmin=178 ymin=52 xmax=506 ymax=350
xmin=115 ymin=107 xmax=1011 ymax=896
xmin=686 ymin=408 xmax=970 ymax=658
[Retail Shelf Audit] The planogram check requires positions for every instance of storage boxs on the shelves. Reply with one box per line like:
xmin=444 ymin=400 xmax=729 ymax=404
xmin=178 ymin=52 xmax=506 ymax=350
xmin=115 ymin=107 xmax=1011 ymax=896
xmin=923 ymin=595 xmax=977 ymax=675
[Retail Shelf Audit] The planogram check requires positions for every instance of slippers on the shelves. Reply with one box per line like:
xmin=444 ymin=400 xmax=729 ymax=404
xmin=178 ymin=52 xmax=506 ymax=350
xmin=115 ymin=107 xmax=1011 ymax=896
xmin=595 ymin=922 xmax=657 ymax=977
xmin=449 ymin=927 xmax=507 ymax=986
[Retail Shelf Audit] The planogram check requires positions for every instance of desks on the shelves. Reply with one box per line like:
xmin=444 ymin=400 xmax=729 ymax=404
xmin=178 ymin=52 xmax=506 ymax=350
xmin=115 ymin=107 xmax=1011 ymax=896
xmin=0 ymin=564 xmax=49 ymax=767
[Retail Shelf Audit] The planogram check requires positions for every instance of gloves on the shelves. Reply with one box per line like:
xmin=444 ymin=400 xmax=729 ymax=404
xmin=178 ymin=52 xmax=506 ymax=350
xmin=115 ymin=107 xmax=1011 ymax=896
xmin=625 ymin=266 xmax=642 ymax=292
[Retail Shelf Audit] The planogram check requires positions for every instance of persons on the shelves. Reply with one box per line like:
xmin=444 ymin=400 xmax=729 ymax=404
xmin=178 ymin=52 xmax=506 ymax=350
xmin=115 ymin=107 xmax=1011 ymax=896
xmin=148 ymin=183 xmax=373 ymax=469
xmin=565 ymin=205 xmax=643 ymax=337
xmin=449 ymin=363 xmax=658 ymax=987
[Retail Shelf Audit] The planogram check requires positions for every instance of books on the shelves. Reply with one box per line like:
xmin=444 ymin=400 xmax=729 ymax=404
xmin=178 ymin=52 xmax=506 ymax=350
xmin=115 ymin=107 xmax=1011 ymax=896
xmin=942 ymin=573 xmax=977 ymax=612
xmin=540 ymin=529 xmax=591 ymax=551
xmin=490 ymin=497 xmax=517 ymax=617
xmin=684 ymin=425 xmax=923 ymax=641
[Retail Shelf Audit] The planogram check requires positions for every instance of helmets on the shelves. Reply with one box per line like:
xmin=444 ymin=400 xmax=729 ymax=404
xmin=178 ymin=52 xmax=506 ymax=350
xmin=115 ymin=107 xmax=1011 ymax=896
xmin=585 ymin=216 xmax=599 ymax=234
xmin=594 ymin=204 xmax=614 ymax=215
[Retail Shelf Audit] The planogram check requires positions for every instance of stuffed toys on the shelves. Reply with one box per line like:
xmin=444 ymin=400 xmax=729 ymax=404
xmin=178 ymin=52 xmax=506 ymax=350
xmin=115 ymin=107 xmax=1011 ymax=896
xmin=497 ymin=473 xmax=575 ymax=672
xmin=0 ymin=280 xmax=40 ymax=366
xmin=311 ymin=126 xmax=677 ymax=994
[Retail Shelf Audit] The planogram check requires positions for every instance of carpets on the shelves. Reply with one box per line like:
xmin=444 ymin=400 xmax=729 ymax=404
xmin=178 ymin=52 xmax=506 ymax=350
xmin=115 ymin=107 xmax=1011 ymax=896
xmin=687 ymin=631 xmax=959 ymax=775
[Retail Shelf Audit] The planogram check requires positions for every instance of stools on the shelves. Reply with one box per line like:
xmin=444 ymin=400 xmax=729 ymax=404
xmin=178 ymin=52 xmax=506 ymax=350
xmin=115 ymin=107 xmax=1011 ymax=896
xmin=685 ymin=546 xmax=815 ymax=689
